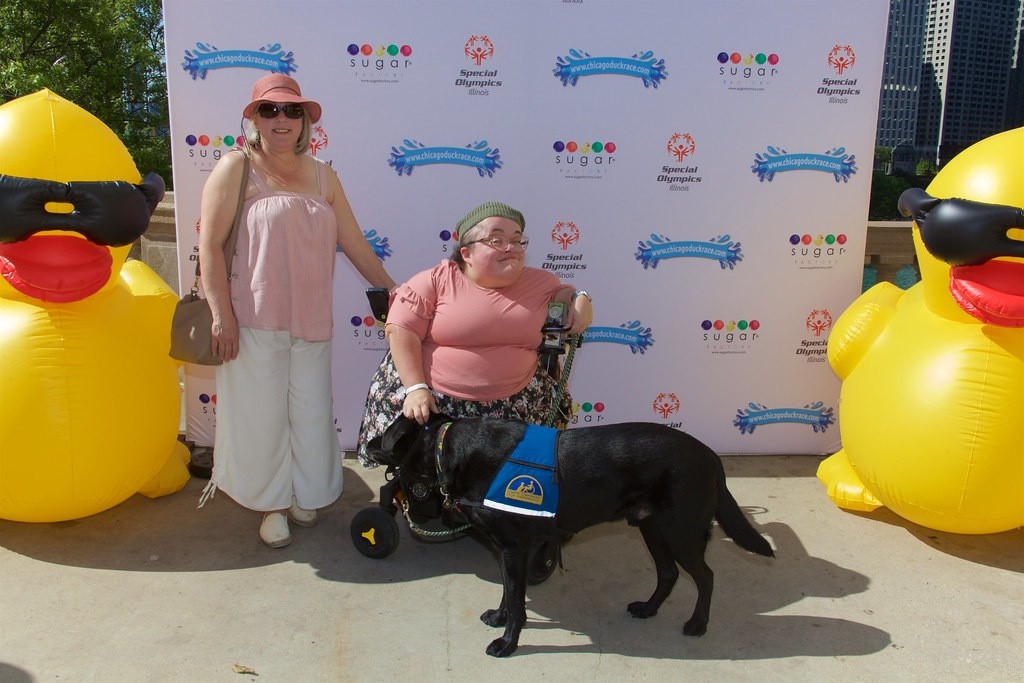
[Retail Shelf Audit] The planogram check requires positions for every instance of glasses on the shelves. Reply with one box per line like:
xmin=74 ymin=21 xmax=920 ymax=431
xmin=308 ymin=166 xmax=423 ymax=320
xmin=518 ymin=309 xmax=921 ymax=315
xmin=465 ymin=236 xmax=529 ymax=252
xmin=258 ymin=103 xmax=304 ymax=119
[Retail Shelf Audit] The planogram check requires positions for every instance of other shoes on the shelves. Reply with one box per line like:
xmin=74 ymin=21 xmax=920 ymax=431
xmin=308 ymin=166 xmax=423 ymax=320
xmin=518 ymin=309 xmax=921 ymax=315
xmin=259 ymin=495 xmax=317 ymax=548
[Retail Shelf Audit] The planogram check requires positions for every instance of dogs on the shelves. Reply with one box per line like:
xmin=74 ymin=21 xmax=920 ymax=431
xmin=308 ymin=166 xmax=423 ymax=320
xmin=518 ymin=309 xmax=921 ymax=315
xmin=391 ymin=406 xmax=777 ymax=661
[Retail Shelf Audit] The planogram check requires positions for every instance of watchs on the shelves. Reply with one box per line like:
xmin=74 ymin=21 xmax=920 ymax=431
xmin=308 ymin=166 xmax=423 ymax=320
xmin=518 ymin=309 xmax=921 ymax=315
xmin=570 ymin=290 xmax=592 ymax=303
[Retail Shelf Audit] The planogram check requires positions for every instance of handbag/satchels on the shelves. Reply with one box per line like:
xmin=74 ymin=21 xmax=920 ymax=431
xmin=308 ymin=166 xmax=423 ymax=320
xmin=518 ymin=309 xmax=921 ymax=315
xmin=170 ymin=294 xmax=226 ymax=367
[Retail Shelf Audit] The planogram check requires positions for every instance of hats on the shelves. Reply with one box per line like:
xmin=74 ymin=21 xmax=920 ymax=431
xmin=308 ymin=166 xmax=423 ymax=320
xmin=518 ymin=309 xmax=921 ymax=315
xmin=456 ymin=202 xmax=526 ymax=243
xmin=244 ymin=74 xmax=322 ymax=126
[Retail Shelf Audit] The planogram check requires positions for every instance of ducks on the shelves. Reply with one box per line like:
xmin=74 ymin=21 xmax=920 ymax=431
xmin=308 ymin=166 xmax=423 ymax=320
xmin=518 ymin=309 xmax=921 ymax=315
xmin=817 ymin=128 xmax=1024 ymax=537
xmin=0 ymin=87 xmax=195 ymax=525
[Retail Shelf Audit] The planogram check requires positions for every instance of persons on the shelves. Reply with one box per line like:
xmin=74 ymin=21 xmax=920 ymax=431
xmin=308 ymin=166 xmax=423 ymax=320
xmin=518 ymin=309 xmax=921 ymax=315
xmin=199 ymin=80 xmax=398 ymax=549
xmin=381 ymin=202 xmax=595 ymax=426
